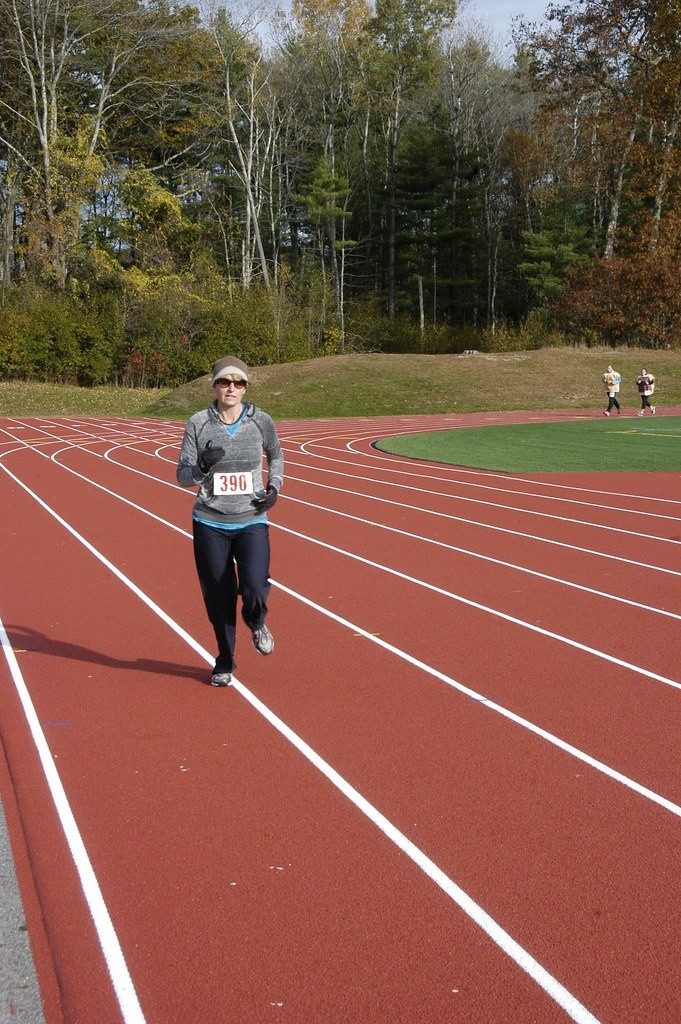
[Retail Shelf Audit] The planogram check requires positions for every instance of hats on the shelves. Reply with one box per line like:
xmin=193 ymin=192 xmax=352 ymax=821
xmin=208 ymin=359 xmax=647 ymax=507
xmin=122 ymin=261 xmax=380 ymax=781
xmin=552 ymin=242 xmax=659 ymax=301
xmin=210 ymin=355 xmax=248 ymax=386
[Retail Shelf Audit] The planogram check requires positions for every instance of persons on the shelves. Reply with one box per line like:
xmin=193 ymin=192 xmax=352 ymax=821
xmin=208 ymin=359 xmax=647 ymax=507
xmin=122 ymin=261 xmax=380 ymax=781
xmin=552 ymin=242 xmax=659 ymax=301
xmin=601 ymin=365 xmax=623 ymax=417
xmin=177 ymin=354 xmax=283 ymax=687
xmin=636 ymin=368 xmax=656 ymax=417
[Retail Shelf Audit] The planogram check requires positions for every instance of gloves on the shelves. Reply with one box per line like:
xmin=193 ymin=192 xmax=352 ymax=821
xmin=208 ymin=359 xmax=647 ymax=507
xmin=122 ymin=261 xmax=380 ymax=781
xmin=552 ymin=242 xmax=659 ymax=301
xmin=250 ymin=485 xmax=277 ymax=516
xmin=198 ymin=440 xmax=226 ymax=476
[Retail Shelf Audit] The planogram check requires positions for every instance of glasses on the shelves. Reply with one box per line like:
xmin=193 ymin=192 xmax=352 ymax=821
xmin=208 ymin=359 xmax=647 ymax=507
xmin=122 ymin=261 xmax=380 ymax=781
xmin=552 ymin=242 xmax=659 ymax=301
xmin=216 ymin=379 xmax=246 ymax=389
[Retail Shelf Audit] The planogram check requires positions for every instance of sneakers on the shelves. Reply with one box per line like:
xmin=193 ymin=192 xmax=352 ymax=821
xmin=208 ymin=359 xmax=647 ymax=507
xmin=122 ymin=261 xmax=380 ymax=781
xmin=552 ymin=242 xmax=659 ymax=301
xmin=210 ymin=672 xmax=233 ymax=687
xmin=241 ymin=615 xmax=274 ymax=656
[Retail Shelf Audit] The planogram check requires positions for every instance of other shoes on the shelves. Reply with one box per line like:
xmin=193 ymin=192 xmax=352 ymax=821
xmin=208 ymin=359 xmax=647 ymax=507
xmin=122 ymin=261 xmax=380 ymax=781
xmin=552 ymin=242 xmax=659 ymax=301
xmin=636 ymin=412 xmax=643 ymax=416
xmin=616 ymin=405 xmax=623 ymax=415
xmin=603 ymin=410 xmax=611 ymax=417
xmin=651 ymin=406 xmax=656 ymax=415
